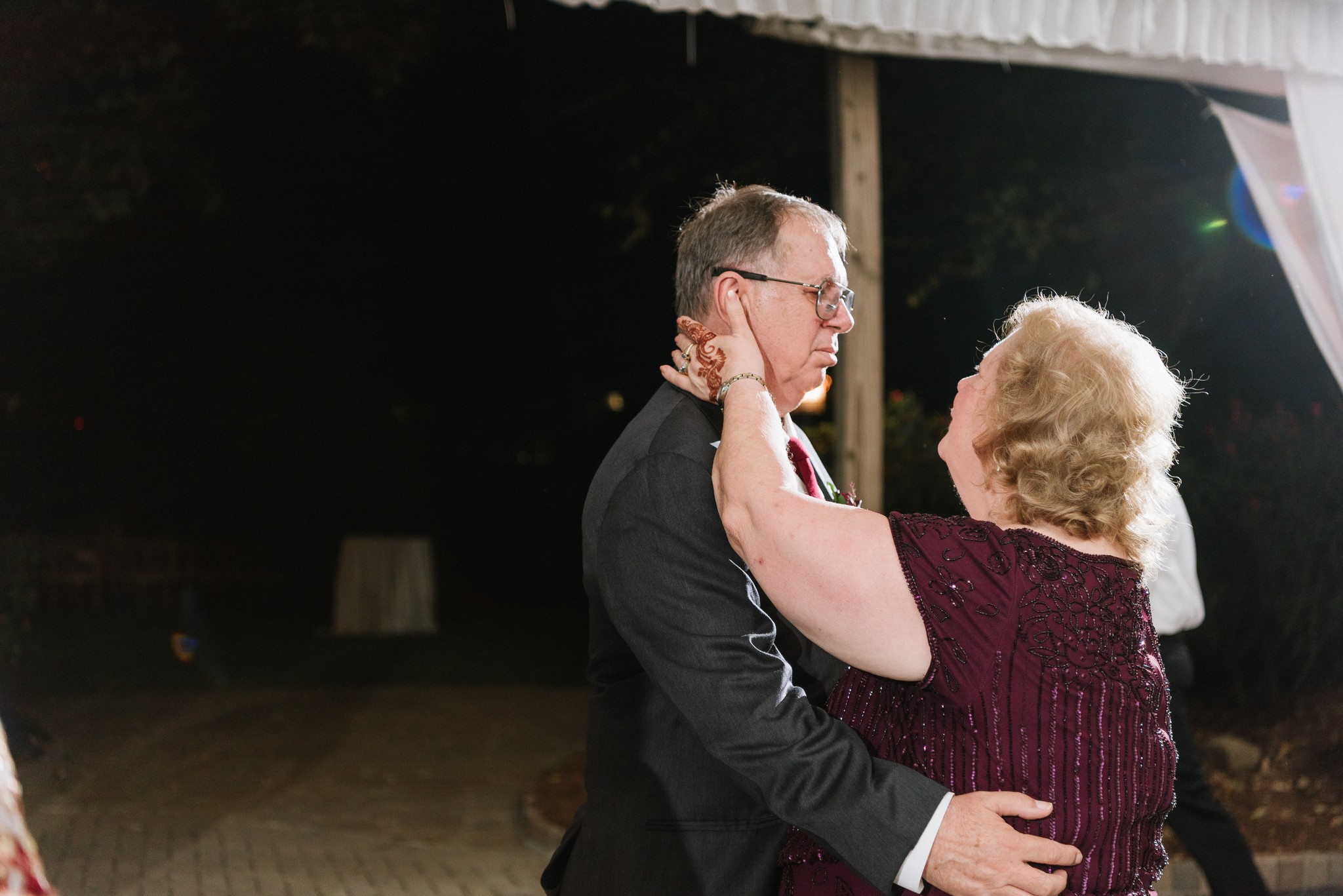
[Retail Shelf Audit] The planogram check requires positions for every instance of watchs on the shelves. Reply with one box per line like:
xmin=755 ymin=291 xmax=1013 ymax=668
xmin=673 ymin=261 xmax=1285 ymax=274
xmin=714 ymin=373 xmax=766 ymax=412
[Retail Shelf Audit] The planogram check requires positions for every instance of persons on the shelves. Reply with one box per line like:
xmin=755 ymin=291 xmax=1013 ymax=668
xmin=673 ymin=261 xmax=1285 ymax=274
xmin=1119 ymin=467 xmax=1282 ymax=895
xmin=656 ymin=284 xmax=1192 ymax=896
xmin=534 ymin=176 xmax=1083 ymax=896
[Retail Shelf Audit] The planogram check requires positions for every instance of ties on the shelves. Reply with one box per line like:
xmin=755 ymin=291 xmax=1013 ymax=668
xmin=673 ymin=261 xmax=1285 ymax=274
xmin=788 ymin=435 xmax=825 ymax=502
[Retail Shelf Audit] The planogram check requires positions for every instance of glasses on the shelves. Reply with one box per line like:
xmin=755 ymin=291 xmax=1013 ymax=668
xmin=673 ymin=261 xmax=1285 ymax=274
xmin=710 ymin=266 xmax=856 ymax=321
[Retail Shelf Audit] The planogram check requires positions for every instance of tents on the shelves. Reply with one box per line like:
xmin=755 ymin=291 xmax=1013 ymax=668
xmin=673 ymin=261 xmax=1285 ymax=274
xmin=577 ymin=1 xmax=1343 ymax=526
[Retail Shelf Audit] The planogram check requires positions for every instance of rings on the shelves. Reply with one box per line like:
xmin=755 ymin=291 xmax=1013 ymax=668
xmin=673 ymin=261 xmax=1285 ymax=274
xmin=681 ymin=342 xmax=695 ymax=360
xmin=678 ymin=361 xmax=689 ymax=376
